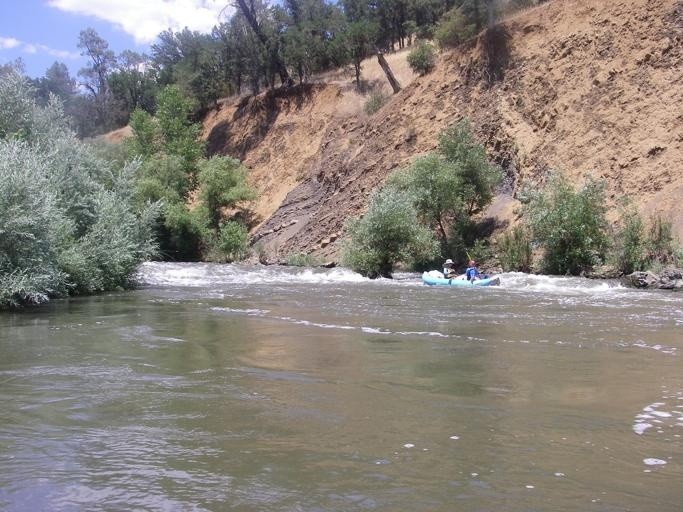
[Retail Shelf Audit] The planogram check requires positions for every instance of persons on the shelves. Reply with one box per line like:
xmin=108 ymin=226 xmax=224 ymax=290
xmin=444 ymin=259 xmax=458 ymax=280
xmin=466 ymin=260 xmax=483 ymax=280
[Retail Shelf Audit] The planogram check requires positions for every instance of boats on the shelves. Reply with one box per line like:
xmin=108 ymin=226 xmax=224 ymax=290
xmin=421 ymin=270 xmax=501 ymax=286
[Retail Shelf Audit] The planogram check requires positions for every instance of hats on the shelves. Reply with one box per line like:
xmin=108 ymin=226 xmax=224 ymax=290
xmin=444 ymin=259 xmax=455 ymax=266
